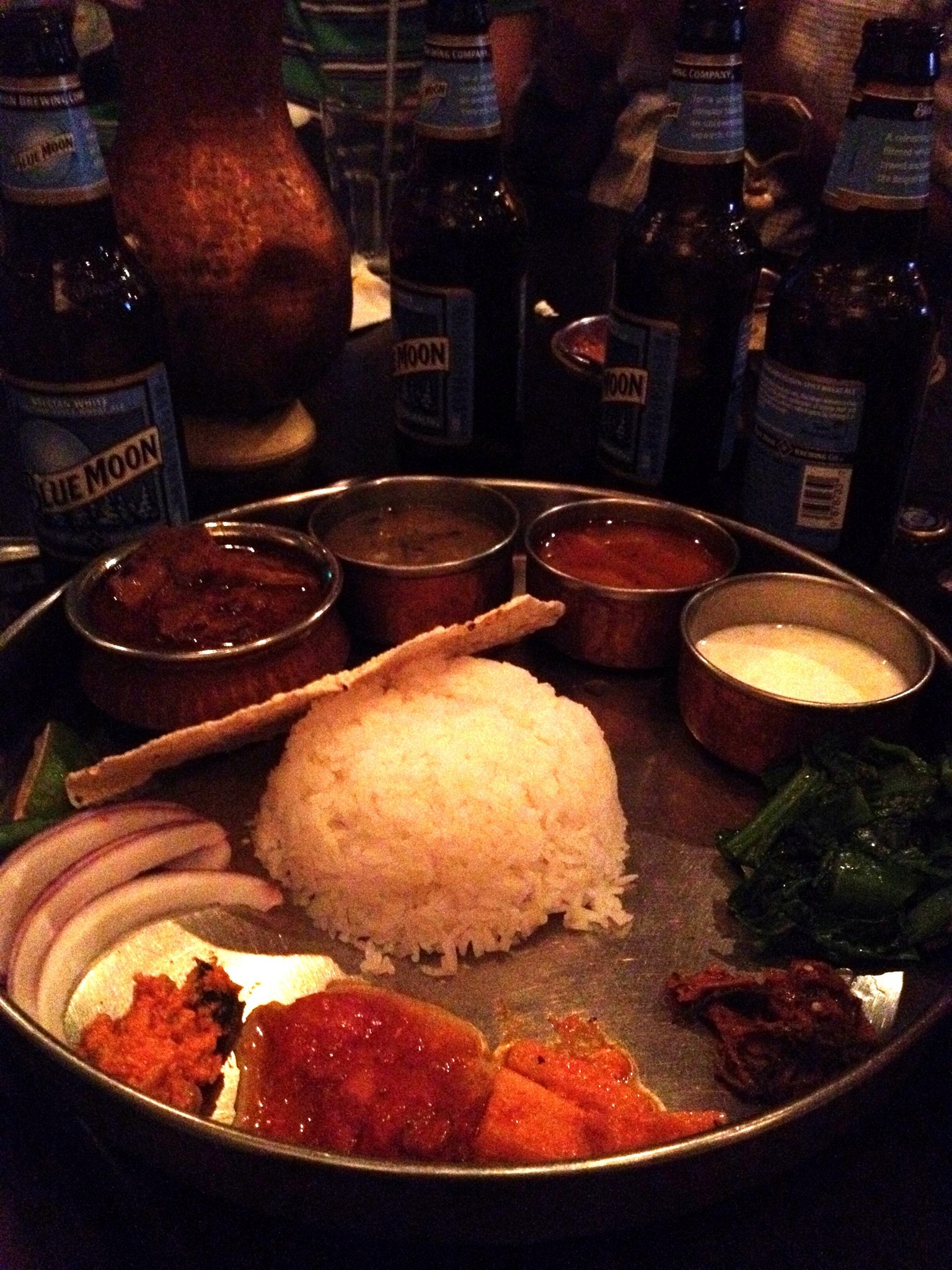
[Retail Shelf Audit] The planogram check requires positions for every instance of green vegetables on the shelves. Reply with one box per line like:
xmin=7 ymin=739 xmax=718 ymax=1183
xmin=715 ymin=724 xmax=952 ymax=978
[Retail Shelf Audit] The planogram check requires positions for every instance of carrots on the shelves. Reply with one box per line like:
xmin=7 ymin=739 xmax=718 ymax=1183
xmin=471 ymin=1042 xmax=731 ymax=1166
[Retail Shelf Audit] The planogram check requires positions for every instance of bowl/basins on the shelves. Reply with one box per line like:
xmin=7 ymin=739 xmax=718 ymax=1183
xmin=307 ymin=479 xmax=524 ymax=649
xmin=517 ymin=501 xmax=745 ymax=674
xmin=672 ymin=571 xmax=939 ymax=792
xmin=64 ymin=517 xmax=348 ymax=752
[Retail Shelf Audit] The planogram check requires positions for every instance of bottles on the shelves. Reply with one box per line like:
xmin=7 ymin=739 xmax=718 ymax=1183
xmin=392 ymin=1 xmax=529 ymax=476
xmin=0 ymin=0 xmax=193 ymax=583
xmin=105 ymin=2 xmax=353 ymax=476
xmin=737 ymin=12 xmax=943 ymax=590
xmin=593 ymin=0 xmax=757 ymax=510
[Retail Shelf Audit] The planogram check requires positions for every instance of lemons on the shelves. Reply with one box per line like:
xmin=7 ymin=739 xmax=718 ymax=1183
xmin=0 ymin=720 xmax=77 ymax=856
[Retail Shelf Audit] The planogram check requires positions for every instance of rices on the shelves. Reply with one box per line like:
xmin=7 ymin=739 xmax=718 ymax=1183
xmin=236 ymin=655 xmax=633 ymax=977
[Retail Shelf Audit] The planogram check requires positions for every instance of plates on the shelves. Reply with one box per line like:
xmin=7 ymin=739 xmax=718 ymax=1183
xmin=0 ymin=467 xmax=952 ymax=1211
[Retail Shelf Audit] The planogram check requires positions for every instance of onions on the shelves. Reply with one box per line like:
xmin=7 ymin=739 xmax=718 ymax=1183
xmin=0 ymin=797 xmax=284 ymax=1042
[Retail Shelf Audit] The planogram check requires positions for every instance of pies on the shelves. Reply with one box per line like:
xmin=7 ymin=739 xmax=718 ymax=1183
xmin=64 ymin=595 xmax=562 ymax=813
